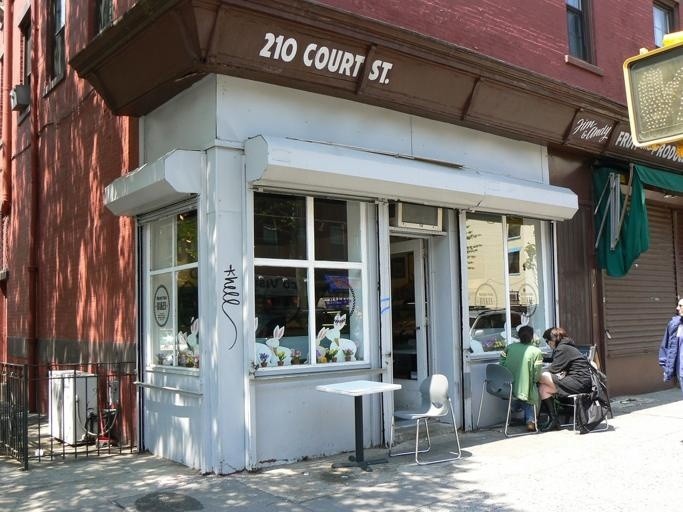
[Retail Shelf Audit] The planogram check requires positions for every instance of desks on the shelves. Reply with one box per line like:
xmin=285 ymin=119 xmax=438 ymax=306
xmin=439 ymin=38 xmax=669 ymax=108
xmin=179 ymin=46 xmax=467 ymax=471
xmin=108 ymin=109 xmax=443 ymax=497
xmin=318 ymin=379 xmax=402 ymax=473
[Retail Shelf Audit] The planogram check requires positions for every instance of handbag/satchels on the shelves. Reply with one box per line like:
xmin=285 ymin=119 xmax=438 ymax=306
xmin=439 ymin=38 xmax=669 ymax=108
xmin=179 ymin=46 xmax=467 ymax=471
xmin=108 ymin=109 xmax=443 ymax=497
xmin=576 ymin=394 xmax=611 ymax=434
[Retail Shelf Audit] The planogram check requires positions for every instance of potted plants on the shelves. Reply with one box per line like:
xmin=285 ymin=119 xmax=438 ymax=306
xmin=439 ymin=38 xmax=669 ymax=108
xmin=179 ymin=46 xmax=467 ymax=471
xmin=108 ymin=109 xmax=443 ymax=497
xmin=152 ymin=350 xmax=354 ymax=367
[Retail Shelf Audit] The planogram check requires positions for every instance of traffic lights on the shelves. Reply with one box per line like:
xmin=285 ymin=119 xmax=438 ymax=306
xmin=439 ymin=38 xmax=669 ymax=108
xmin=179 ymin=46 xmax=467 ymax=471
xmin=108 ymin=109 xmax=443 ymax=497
xmin=622 ymin=31 xmax=683 ymax=148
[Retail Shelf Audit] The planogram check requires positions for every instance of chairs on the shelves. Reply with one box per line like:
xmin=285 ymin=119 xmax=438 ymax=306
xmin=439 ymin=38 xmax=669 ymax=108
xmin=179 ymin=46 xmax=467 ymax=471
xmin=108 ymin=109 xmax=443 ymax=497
xmin=554 ymin=362 xmax=610 ymax=433
xmin=473 ymin=364 xmax=538 ymax=436
xmin=389 ymin=374 xmax=462 ymax=463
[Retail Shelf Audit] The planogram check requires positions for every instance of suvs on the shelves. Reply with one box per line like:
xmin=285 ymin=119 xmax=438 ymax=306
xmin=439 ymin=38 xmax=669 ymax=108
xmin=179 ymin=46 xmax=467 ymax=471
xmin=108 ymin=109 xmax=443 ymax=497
xmin=469 ymin=304 xmax=530 ymax=343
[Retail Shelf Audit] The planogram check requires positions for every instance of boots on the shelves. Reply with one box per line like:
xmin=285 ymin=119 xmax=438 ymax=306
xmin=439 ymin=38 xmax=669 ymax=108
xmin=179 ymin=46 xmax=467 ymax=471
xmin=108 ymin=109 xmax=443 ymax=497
xmin=541 ymin=396 xmax=561 ymax=432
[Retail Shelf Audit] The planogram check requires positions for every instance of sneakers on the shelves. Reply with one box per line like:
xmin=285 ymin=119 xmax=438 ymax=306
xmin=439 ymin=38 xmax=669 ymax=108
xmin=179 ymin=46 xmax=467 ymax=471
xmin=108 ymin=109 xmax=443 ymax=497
xmin=527 ymin=423 xmax=535 ymax=431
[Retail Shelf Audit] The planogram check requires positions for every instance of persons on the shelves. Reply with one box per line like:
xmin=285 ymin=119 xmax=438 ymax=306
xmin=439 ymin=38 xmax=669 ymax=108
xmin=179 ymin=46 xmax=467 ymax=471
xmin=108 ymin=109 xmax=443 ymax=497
xmin=659 ymin=298 xmax=683 ymax=395
xmin=499 ymin=325 xmax=593 ymax=433
